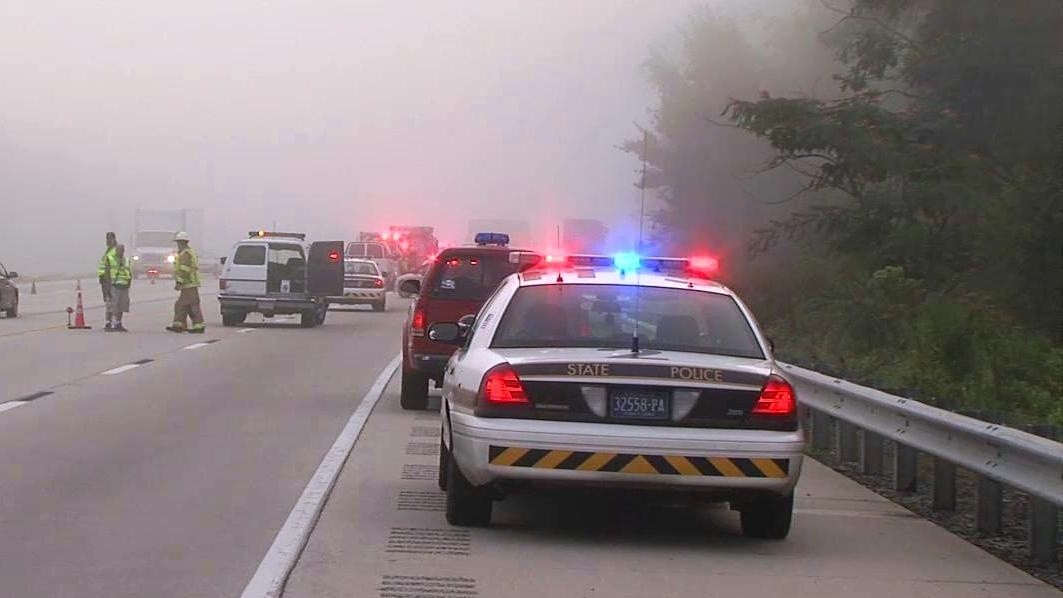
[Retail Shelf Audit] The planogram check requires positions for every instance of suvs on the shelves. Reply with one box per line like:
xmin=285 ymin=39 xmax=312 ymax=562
xmin=216 ymin=230 xmax=346 ymax=328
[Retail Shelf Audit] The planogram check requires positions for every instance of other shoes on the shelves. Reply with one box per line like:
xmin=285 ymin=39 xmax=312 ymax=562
xmin=166 ymin=326 xmax=183 ymax=333
xmin=105 ymin=324 xmax=117 ymax=332
xmin=189 ymin=329 xmax=204 ymax=334
xmin=114 ymin=324 xmax=128 ymax=332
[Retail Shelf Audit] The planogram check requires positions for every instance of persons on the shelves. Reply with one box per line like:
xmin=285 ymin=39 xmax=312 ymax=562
xmin=165 ymin=231 xmax=205 ymax=333
xmin=96 ymin=232 xmax=117 ymax=302
xmin=104 ymin=243 xmax=133 ymax=332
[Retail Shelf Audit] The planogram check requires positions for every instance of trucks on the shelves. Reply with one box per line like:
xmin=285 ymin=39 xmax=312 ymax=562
xmin=128 ymin=228 xmax=178 ymax=277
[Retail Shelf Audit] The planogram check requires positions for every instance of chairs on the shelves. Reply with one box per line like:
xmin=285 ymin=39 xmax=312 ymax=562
xmin=527 ymin=307 xmax=575 ymax=343
xmin=359 ymin=265 xmax=370 ymax=273
xmin=659 ymin=314 xmax=702 ymax=349
xmin=286 ymin=257 xmax=304 ymax=273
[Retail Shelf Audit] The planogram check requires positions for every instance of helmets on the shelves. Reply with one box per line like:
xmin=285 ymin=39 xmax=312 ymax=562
xmin=172 ymin=231 xmax=190 ymax=240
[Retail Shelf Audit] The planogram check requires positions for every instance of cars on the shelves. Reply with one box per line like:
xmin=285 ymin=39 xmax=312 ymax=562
xmin=0 ymin=263 xmax=20 ymax=318
xmin=396 ymin=231 xmax=536 ymax=412
xmin=437 ymin=252 xmax=807 ymax=540
xmin=323 ymin=224 xmax=440 ymax=310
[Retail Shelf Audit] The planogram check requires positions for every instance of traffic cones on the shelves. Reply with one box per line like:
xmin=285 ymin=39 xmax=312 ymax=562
xmin=30 ymin=282 xmax=37 ymax=295
xmin=68 ymin=292 xmax=93 ymax=329
